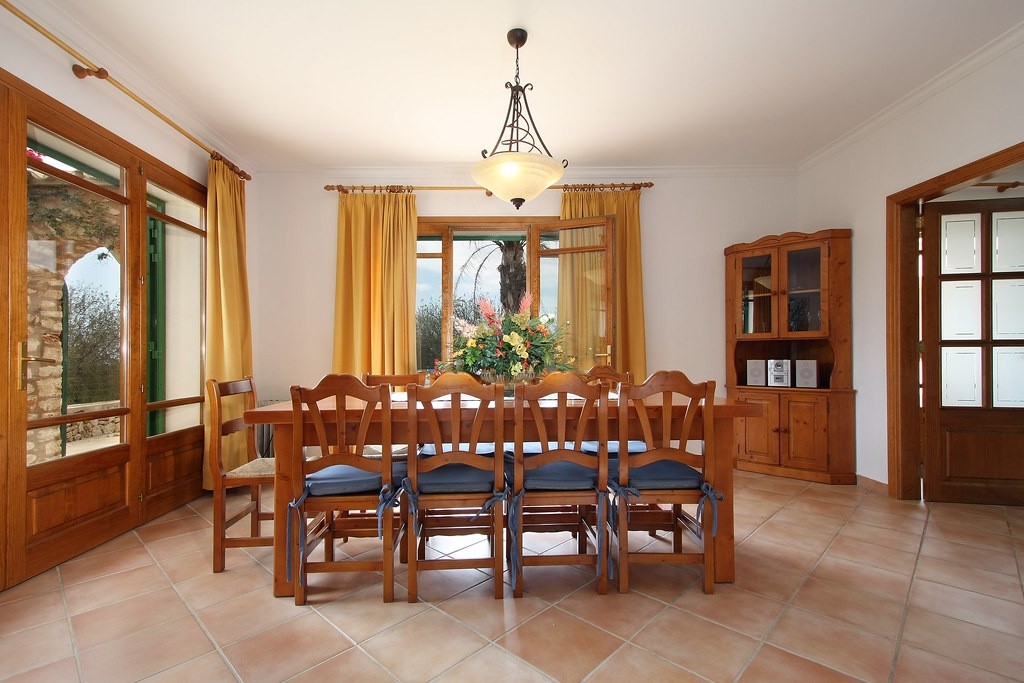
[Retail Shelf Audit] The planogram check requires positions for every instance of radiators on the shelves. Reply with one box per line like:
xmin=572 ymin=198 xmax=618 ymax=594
xmin=255 ymin=400 xmax=287 ymax=459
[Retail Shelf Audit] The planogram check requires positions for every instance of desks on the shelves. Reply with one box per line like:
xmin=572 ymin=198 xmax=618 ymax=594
xmin=244 ymin=392 xmax=762 ymax=597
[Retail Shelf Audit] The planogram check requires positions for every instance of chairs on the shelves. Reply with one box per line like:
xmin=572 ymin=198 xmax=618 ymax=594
xmin=290 ymin=369 xmax=716 ymax=605
xmin=206 ymin=375 xmax=317 ymax=573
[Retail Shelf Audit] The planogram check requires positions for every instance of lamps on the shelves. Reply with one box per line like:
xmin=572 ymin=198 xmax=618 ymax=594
xmin=470 ymin=28 xmax=569 ymax=210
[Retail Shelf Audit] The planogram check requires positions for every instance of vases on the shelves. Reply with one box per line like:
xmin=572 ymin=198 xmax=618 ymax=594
xmin=480 ymin=370 xmax=538 ymax=398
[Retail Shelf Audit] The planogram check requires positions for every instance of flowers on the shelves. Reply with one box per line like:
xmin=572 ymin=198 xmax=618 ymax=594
xmin=434 ymin=293 xmax=586 ymax=383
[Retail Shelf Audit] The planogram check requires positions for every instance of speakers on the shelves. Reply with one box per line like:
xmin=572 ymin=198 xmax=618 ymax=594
xmin=796 ymin=360 xmax=817 ymax=388
xmin=747 ymin=360 xmax=766 ymax=387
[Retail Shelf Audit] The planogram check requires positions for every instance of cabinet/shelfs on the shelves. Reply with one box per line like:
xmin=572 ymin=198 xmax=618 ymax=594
xmin=723 ymin=228 xmax=857 ymax=486
